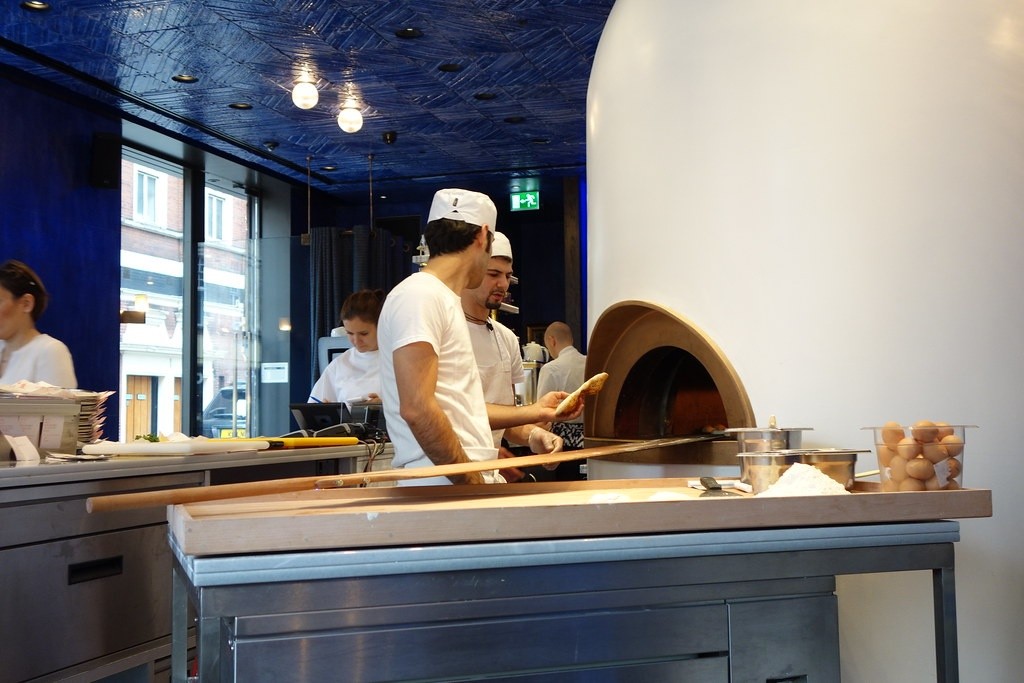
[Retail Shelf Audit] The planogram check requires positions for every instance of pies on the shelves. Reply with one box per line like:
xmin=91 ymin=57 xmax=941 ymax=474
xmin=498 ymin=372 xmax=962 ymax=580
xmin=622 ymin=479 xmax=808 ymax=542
xmin=556 ymin=372 xmax=608 ymax=416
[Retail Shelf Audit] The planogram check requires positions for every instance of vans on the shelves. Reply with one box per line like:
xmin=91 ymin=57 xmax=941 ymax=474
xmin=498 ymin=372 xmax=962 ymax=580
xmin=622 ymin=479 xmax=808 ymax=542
xmin=202 ymin=381 xmax=246 ymax=438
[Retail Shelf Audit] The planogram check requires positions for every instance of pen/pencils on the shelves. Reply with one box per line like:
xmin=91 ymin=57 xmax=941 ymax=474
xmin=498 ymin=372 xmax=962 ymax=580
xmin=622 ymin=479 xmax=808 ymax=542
xmin=309 ymin=396 xmax=323 ymax=403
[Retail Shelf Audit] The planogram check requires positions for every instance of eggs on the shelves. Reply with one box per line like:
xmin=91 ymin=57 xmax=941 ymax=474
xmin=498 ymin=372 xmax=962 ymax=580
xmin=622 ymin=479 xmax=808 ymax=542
xmin=877 ymin=420 xmax=963 ymax=492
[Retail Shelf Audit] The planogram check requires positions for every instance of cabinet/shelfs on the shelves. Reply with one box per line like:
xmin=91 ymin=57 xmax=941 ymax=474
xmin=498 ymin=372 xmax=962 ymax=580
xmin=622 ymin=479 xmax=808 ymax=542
xmin=1 ymin=445 xmax=389 ymax=682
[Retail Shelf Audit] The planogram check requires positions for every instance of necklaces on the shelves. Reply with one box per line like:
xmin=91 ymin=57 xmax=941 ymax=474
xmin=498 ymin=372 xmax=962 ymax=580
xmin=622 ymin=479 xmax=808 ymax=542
xmin=464 ymin=312 xmax=494 ymax=332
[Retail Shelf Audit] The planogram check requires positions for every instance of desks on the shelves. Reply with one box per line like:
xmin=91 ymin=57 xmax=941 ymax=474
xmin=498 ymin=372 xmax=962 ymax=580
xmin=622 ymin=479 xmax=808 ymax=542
xmin=159 ymin=518 xmax=962 ymax=683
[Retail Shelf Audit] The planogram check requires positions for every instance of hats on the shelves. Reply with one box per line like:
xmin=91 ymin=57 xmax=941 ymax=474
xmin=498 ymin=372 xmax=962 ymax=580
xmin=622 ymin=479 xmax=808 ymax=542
xmin=491 ymin=232 xmax=513 ymax=260
xmin=427 ymin=188 xmax=497 ymax=236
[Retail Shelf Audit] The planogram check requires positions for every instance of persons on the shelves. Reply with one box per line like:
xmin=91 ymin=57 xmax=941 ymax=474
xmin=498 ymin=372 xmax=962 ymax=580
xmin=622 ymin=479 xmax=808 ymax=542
xmin=0 ymin=258 xmax=79 ymax=391
xmin=306 ymin=288 xmax=383 ymax=415
xmin=377 ymin=188 xmax=506 ymax=488
xmin=461 ymin=230 xmax=563 ymax=482
xmin=536 ymin=321 xmax=587 ymax=481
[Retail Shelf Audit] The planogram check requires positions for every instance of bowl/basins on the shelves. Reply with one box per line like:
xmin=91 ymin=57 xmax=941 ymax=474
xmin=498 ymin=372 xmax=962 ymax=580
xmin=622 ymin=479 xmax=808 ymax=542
xmin=736 ymin=448 xmax=872 ymax=495
xmin=723 ymin=427 xmax=816 ymax=483
xmin=860 ymin=425 xmax=982 ymax=493
